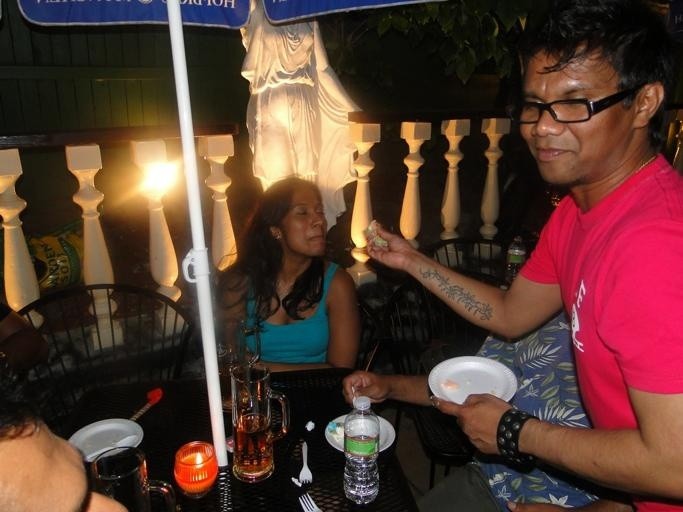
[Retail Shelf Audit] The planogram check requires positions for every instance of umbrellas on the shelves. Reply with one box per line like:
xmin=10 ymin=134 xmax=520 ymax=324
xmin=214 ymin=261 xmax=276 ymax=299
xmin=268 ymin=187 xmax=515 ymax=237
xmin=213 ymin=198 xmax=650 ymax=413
xmin=14 ymin=0 xmax=449 ymax=511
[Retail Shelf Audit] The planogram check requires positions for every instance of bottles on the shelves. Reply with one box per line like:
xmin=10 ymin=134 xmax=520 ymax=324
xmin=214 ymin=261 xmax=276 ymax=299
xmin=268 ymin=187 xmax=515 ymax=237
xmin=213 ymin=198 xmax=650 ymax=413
xmin=343 ymin=396 xmax=380 ymax=505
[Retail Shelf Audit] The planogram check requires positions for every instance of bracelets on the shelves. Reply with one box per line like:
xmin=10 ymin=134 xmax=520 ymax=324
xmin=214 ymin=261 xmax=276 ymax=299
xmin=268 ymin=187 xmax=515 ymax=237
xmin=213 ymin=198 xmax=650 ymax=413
xmin=496 ymin=407 xmax=540 ymax=465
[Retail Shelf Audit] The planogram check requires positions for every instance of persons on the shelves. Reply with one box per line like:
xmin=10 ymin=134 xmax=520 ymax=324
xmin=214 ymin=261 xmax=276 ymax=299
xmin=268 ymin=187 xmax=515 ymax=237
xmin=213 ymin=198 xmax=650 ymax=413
xmin=0 ymin=300 xmax=50 ymax=372
xmin=359 ymin=0 xmax=683 ymax=511
xmin=1 ymin=400 xmax=132 ymax=511
xmin=210 ymin=174 xmax=359 ymax=374
xmin=339 ymin=278 xmax=607 ymax=512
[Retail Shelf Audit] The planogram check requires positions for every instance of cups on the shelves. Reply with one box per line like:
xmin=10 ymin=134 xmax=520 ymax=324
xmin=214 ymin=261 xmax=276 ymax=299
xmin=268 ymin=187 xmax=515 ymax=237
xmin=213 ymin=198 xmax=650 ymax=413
xmin=91 ymin=446 xmax=181 ymax=512
xmin=230 ymin=363 xmax=291 ymax=484
xmin=215 ymin=316 xmax=261 ymax=412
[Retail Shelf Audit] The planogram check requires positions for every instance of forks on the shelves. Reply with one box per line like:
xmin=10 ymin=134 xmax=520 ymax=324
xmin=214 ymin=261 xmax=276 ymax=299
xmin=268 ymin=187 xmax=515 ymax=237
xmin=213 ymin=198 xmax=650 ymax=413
xmin=298 ymin=441 xmax=313 ymax=485
xmin=298 ymin=493 xmax=324 ymax=512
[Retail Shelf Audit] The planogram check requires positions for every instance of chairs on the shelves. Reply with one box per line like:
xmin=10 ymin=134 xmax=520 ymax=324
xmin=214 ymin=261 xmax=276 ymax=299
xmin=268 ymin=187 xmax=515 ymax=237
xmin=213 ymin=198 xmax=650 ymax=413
xmin=358 ymin=300 xmax=406 ymax=449
xmin=393 ymin=238 xmax=507 ymax=490
xmin=1 ymin=283 xmax=194 ymax=441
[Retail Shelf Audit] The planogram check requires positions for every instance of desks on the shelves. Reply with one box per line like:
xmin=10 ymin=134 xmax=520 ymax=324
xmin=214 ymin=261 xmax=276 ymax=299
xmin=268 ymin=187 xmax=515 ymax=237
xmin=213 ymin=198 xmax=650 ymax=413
xmin=62 ymin=368 xmax=420 ymax=512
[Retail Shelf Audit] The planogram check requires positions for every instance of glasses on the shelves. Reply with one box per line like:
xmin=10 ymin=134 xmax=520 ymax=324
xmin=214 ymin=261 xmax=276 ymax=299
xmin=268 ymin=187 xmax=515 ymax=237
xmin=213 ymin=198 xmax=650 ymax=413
xmin=505 ymin=81 xmax=644 ymax=124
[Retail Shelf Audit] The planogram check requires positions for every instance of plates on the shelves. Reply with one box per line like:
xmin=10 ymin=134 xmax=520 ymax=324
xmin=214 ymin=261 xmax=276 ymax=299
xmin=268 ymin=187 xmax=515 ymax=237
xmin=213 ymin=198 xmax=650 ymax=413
xmin=66 ymin=419 xmax=144 ymax=463
xmin=427 ymin=356 xmax=519 ymax=404
xmin=325 ymin=413 xmax=396 ymax=454
